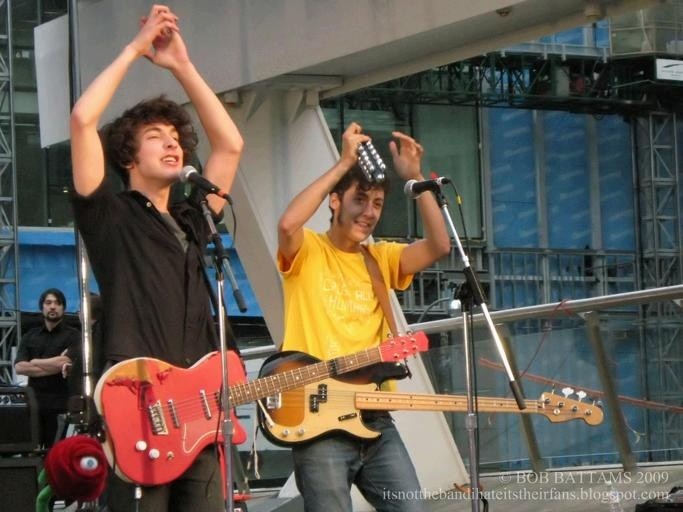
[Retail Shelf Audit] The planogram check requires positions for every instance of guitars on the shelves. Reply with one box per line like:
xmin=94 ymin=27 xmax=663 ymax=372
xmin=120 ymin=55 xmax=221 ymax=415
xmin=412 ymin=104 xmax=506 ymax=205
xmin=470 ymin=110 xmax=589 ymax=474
xmin=94 ymin=330 xmax=429 ymax=486
xmin=256 ymin=351 xmax=605 ymax=448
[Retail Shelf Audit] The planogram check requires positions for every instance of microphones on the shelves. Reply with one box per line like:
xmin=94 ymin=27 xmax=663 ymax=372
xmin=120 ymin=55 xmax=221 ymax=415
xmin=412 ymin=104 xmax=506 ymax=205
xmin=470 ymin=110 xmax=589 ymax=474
xmin=403 ymin=176 xmax=448 ymax=200
xmin=178 ymin=165 xmax=230 ymax=199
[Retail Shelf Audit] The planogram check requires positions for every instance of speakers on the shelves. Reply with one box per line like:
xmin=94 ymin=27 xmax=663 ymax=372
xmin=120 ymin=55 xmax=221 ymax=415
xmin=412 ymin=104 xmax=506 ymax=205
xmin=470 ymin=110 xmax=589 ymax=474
xmin=0 ymin=457 xmax=44 ymax=512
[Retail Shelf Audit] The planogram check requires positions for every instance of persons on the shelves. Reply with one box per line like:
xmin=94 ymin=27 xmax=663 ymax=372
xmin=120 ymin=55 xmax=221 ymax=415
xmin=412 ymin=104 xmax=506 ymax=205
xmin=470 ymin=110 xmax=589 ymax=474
xmin=12 ymin=288 xmax=83 ymax=449
xmin=68 ymin=0 xmax=243 ymax=511
xmin=275 ymin=118 xmax=456 ymax=512
xmin=59 ymin=291 xmax=108 ymax=397
